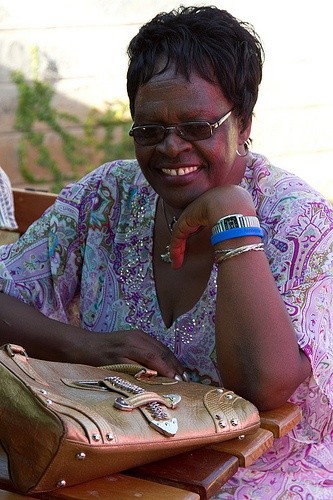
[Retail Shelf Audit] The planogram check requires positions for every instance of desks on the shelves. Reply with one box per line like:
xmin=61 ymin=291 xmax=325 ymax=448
xmin=0 ymin=400 xmax=303 ymax=496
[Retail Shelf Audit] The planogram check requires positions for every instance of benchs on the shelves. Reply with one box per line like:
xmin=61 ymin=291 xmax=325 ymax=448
xmin=0 ymin=189 xmax=61 ymax=243
